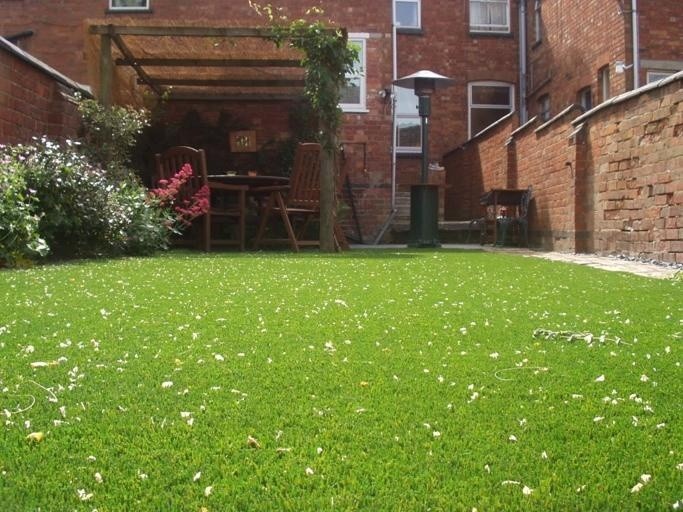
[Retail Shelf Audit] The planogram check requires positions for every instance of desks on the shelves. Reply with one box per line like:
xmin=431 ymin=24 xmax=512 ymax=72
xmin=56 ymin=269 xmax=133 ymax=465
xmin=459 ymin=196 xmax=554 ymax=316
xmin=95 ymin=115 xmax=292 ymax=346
xmin=191 ymin=174 xmax=300 ymax=252
xmin=479 ymin=186 xmax=531 ymax=249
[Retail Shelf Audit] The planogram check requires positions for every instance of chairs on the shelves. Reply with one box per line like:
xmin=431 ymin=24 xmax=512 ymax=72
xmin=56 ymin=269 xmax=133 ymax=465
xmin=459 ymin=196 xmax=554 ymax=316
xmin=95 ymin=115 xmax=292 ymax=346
xmin=154 ymin=145 xmax=249 ymax=252
xmin=255 ymin=141 xmax=342 ymax=252
